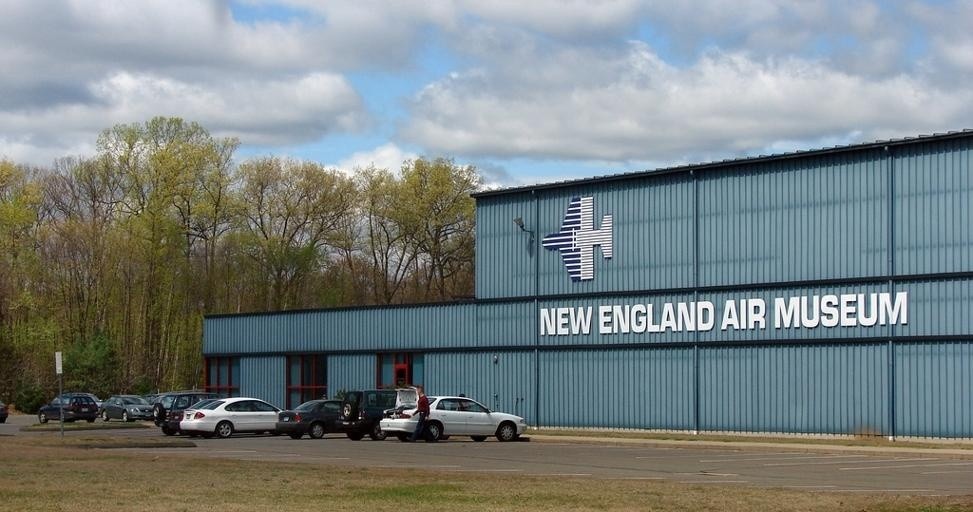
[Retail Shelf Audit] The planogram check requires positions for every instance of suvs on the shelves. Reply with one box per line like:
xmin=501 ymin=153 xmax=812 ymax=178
xmin=337 ymin=387 xmax=421 ymax=442
xmin=151 ymin=392 xmax=234 ymax=440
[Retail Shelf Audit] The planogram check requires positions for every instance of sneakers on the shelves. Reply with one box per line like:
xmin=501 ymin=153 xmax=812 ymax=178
xmin=406 ymin=437 xmax=416 ymax=442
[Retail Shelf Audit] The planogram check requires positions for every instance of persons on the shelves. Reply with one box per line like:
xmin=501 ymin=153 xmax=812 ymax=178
xmin=457 ymin=394 xmax=472 ymax=411
xmin=406 ymin=384 xmax=436 ymax=442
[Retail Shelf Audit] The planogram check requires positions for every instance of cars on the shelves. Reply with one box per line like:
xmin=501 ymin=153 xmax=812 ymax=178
xmin=177 ymin=394 xmax=287 ymax=440
xmin=0 ymin=400 xmax=8 ymax=423
xmin=274 ymin=397 xmax=346 ymax=440
xmin=377 ymin=386 xmax=529 ymax=444
xmin=37 ymin=392 xmax=164 ymax=424
